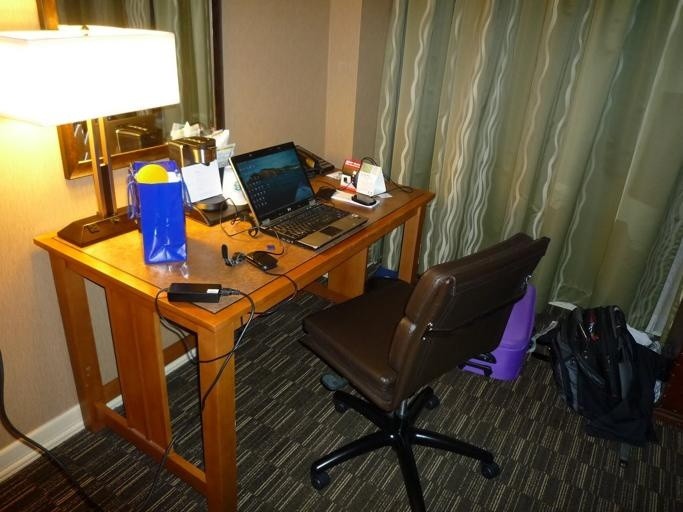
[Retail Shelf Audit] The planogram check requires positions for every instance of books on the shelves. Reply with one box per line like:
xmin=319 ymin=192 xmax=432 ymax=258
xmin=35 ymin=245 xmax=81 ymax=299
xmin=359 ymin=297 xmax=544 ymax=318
xmin=330 ymin=189 xmax=380 ymax=210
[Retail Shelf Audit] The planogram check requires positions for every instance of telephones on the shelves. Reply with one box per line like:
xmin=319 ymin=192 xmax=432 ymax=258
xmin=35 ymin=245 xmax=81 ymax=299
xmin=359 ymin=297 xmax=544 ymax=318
xmin=295 ymin=145 xmax=334 ymax=174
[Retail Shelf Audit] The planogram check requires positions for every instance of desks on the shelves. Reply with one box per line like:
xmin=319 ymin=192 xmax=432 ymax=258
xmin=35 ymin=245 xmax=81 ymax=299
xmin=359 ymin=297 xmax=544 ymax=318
xmin=33 ymin=164 xmax=435 ymax=511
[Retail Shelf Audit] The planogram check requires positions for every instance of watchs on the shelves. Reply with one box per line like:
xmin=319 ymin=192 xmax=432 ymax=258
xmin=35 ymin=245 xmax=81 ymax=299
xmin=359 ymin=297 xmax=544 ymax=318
xmin=219 ymin=245 xmax=246 ymax=268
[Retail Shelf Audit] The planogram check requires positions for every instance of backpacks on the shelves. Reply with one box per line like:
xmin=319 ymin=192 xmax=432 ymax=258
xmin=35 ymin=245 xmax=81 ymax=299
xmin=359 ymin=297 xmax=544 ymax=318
xmin=551 ymin=300 xmax=659 ymax=446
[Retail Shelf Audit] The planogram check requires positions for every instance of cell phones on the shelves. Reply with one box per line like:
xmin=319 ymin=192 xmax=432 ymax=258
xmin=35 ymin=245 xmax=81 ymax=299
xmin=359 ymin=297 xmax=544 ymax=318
xmin=351 ymin=194 xmax=376 ymax=206
xmin=245 ymin=251 xmax=279 ymax=270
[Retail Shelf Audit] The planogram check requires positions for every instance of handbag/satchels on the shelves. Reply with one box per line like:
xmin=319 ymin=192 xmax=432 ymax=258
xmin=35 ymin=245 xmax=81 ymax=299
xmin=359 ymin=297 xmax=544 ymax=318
xmin=127 ymin=159 xmax=188 ymax=266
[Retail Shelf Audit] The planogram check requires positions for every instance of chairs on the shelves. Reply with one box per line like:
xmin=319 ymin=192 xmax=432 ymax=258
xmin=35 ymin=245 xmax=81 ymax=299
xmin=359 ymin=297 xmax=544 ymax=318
xmin=296 ymin=231 xmax=551 ymax=512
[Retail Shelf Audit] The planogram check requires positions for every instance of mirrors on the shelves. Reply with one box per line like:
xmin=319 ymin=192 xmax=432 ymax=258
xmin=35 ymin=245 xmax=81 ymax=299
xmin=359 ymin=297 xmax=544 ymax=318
xmin=35 ymin=0 xmax=226 ymax=180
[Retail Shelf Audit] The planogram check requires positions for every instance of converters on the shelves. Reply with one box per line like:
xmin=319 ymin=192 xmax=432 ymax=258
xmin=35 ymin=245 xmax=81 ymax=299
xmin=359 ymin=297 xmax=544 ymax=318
xmin=167 ymin=283 xmax=222 ymax=303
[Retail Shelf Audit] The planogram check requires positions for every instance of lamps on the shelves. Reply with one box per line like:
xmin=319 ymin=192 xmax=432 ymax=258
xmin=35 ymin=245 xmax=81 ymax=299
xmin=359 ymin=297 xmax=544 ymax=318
xmin=0 ymin=25 xmax=182 ymax=247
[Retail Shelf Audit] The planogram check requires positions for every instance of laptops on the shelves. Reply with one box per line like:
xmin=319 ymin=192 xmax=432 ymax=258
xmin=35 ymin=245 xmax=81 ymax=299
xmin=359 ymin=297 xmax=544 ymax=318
xmin=229 ymin=141 xmax=368 ymax=251
